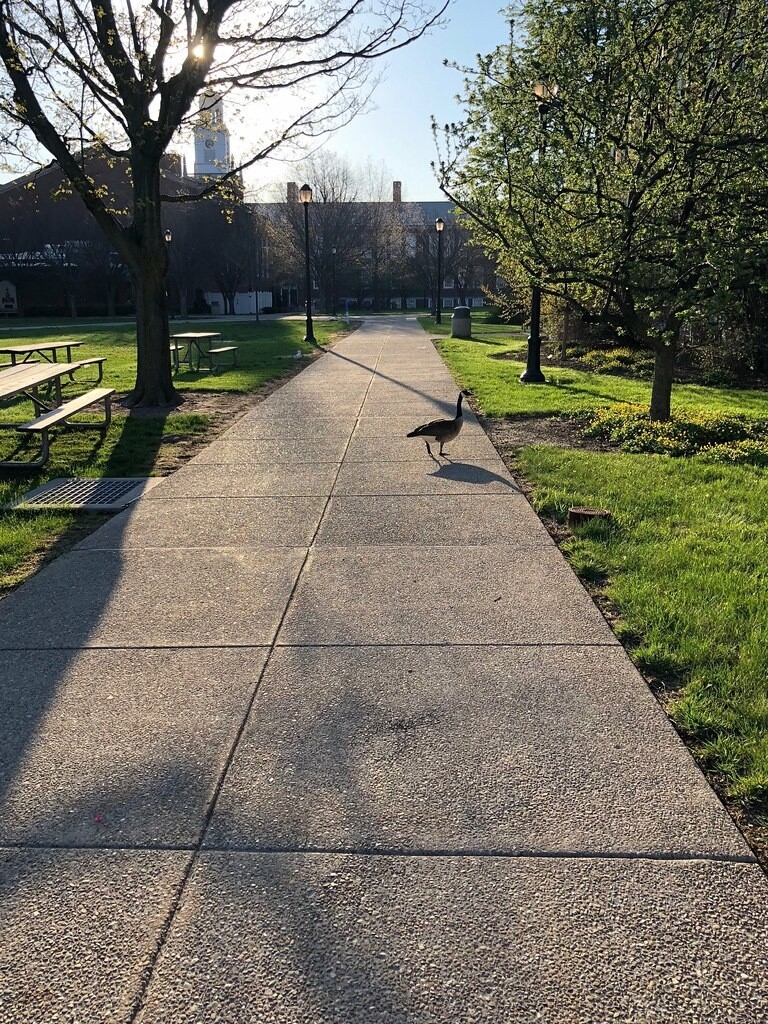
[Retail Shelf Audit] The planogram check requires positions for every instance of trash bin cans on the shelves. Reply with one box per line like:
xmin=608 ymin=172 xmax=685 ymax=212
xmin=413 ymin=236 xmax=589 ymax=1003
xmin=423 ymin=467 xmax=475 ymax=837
xmin=305 ymin=301 xmax=315 ymax=315
xmin=451 ymin=306 xmax=471 ymax=338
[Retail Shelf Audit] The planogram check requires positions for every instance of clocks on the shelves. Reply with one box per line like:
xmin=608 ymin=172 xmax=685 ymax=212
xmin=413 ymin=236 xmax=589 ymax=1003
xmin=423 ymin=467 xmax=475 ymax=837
xmin=205 ymin=139 xmax=214 ymax=149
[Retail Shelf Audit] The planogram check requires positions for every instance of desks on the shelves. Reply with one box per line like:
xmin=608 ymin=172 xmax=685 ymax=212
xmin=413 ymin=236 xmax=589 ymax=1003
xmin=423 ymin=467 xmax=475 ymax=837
xmin=0 ymin=363 xmax=80 ymax=468
xmin=0 ymin=342 xmax=85 ymax=382
xmin=169 ymin=332 xmax=221 ymax=373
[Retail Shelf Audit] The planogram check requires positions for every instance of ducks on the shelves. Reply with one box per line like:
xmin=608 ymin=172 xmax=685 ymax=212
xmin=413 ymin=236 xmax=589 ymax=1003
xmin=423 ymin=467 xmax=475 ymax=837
xmin=407 ymin=389 xmax=474 ymax=455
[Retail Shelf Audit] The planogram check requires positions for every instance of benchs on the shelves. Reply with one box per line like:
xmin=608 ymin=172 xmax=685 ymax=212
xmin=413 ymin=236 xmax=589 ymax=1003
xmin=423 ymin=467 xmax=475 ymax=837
xmin=170 ymin=345 xmax=185 ymax=370
xmin=0 ymin=359 xmax=40 ymax=372
xmin=69 ymin=358 xmax=107 ymax=385
xmin=15 ymin=388 xmax=116 ymax=467
xmin=206 ymin=347 xmax=239 ymax=371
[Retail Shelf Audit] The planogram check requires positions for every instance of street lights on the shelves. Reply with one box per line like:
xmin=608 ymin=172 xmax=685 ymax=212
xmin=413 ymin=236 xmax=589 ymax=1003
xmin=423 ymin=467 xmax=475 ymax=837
xmin=300 ymin=184 xmax=317 ymax=341
xmin=434 ymin=217 xmax=446 ymax=325
xmin=164 ymin=229 xmax=179 ymax=320
xmin=520 ymin=81 xmax=558 ymax=379
